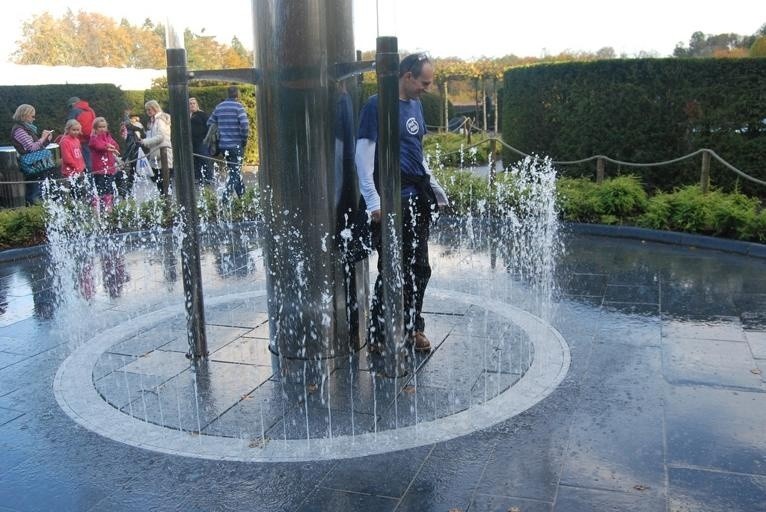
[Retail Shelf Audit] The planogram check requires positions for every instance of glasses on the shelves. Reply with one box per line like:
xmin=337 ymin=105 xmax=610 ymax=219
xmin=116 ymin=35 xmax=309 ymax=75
xmin=406 ymin=50 xmax=430 ymax=73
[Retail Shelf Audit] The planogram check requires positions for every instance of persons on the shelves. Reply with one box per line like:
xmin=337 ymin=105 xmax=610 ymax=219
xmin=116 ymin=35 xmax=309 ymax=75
xmin=9 ymin=96 xmax=214 ymax=207
xmin=204 ymin=86 xmax=250 ymax=207
xmin=353 ymin=54 xmax=449 ymax=355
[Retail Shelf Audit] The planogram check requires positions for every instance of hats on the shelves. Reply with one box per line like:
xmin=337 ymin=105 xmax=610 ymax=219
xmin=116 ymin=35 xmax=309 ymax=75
xmin=66 ymin=97 xmax=80 ymax=110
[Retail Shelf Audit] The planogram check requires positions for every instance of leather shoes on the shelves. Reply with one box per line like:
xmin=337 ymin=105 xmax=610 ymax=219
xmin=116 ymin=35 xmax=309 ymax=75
xmin=405 ymin=331 xmax=431 ymax=351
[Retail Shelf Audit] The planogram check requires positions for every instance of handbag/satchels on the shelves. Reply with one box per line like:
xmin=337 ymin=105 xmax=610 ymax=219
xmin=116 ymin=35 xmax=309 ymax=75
xmin=17 ymin=148 xmax=55 ymax=177
